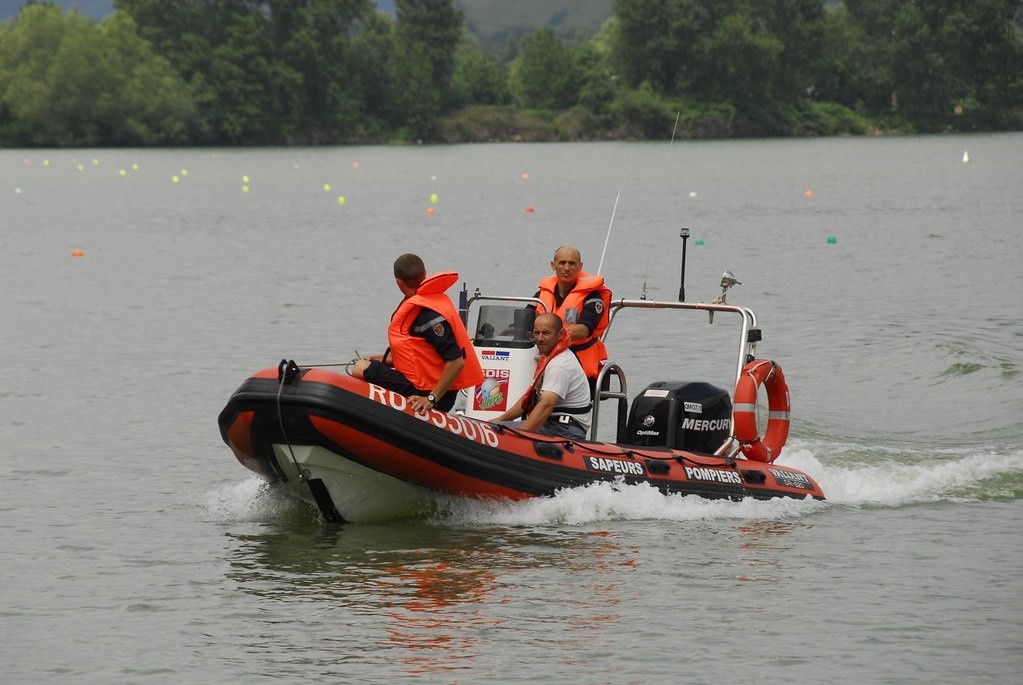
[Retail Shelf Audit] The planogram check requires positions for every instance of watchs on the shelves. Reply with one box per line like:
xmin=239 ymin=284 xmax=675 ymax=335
xmin=427 ymin=394 xmax=438 ymax=405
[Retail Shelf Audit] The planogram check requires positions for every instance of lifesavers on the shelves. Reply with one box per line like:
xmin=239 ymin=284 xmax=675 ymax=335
xmin=732 ymin=358 xmax=791 ymax=463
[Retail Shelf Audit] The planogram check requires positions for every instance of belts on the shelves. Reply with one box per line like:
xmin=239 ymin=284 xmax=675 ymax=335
xmin=548 ymin=416 xmax=585 ymax=431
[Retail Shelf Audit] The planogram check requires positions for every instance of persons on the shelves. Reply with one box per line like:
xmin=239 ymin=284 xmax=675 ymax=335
xmin=352 ymin=254 xmax=484 ymax=416
xmin=526 ymin=245 xmax=612 ymax=401
xmin=489 ymin=313 xmax=592 ymax=441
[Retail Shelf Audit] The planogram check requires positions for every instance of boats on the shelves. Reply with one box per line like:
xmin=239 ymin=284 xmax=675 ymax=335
xmin=217 ymin=226 xmax=828 ymax=524
